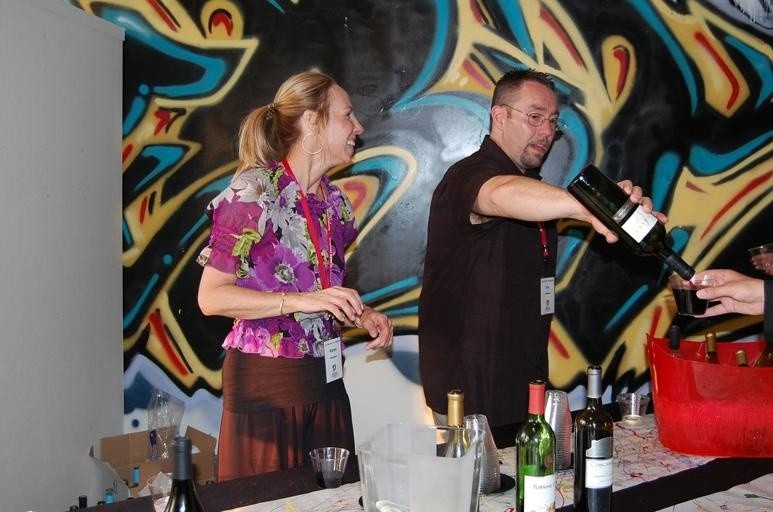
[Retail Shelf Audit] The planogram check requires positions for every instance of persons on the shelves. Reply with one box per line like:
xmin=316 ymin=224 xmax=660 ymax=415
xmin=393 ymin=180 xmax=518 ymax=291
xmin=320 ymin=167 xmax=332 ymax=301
xmin=679 ymin=264 xmax=772 ymax=323
xmin=419 ymin=68 xmax=668 ymax=446
xmin=195 ymin=71 xmax=395 ymax=477
xmin=753 ymin=248 xmax=773 ymax=270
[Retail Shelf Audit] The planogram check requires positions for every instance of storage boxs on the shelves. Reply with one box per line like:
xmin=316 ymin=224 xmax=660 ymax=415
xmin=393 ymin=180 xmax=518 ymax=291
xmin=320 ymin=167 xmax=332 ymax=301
xmin=90 ymin=425 xmax=217 ymax=500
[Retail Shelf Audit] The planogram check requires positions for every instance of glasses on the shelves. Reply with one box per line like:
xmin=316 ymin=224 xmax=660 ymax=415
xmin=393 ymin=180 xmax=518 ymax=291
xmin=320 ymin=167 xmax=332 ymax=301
xmin=498 ymin=103 xmax=568 ymax=131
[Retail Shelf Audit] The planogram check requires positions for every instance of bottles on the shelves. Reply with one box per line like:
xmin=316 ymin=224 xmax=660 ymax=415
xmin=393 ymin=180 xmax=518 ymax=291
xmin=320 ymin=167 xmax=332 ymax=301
xmin=132 ymin=464 xmax=140 ymax=488
xmin=704 ymin=333 xmax=720 ymax=364
xmin=568 ymin=163 xmax=695 ymax=278
xmin=667 ymin=326 xmax=684 ymax=359
xmin=104 ymin=488 xmax=116 ymax=504
xmin=573 ymin=367 xmax=612 ymax=511
xmin=163 ymin=437 xmax=202 ymax=512
xmin=736 ymin=350 xmax=749 ymax=368
xmin=446 ymin=389 xmax=470 ymax=459
xmin=78 ymin=495 xmax=88 ymax=509
xmin=516 ymin=380 xmax=555 ymax=512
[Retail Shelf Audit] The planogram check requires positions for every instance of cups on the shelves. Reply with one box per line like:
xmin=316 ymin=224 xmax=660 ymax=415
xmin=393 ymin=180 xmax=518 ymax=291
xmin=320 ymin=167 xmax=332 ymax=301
xmin=355 ymin=422 xmax=482 ymax=512
xmin=747 ymin=244 xmax=773 ymax=274
xmin=146 ymin=471 xmax=174 ymax=511
xmin=309 ymin=447 xmax=349 ymax=492
xmin=463 ymin=412 xmax=501 ymax=496
xmin=669 ymin=273 xmax=715 ymax=317
xmin=619 ymin=393 xmax=650 ymax=427
xmin=542 ymin=389 xmax=573 ymax=471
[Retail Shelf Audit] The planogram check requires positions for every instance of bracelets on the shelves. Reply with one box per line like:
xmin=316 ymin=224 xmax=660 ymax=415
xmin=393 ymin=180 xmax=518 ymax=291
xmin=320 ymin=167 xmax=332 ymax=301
xmin=278 ymin=292 xmax=285 ymax=318
